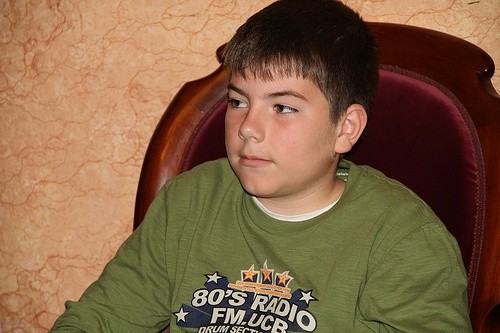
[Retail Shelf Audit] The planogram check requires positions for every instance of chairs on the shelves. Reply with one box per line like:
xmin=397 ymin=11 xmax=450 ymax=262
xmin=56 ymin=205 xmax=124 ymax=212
xmin=133 ymin=21 xmax=500 ymax=333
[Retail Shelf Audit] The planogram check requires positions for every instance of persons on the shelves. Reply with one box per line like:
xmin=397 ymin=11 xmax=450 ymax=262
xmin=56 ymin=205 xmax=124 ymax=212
xmin=49 ymin=0 xmax=473 ymax=333
